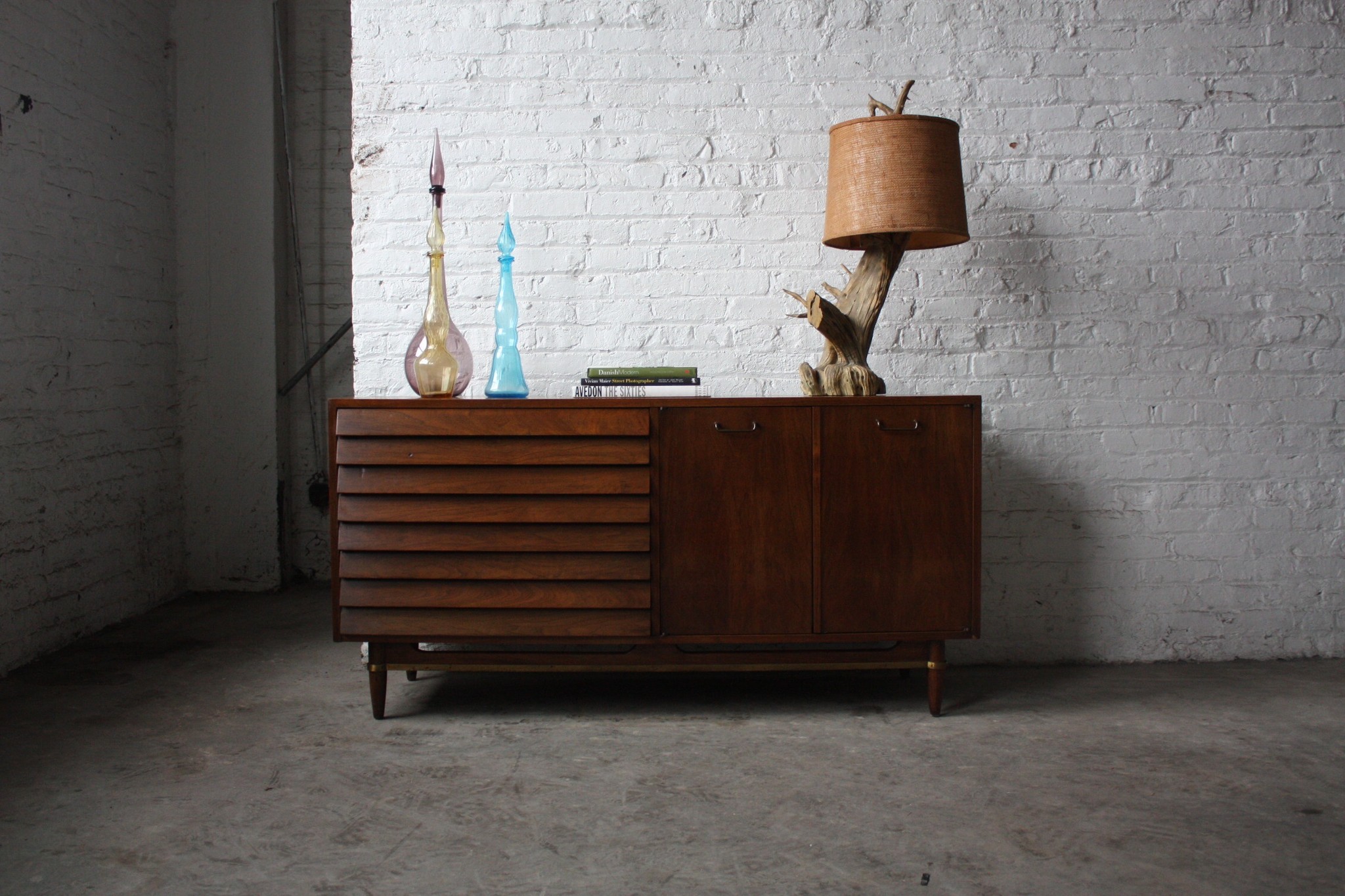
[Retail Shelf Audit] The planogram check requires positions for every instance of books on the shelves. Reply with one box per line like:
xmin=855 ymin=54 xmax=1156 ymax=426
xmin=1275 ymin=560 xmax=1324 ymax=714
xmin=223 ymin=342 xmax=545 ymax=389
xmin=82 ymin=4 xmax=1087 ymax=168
xmin=572 ymin=367 xmax=711 ymax=399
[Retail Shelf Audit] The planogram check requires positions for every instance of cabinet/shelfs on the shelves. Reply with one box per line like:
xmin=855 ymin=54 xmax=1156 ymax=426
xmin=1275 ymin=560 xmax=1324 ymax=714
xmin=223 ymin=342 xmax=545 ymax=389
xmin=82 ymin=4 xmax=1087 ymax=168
xmin=322 ymin=395 xmax=981 ymax=718
